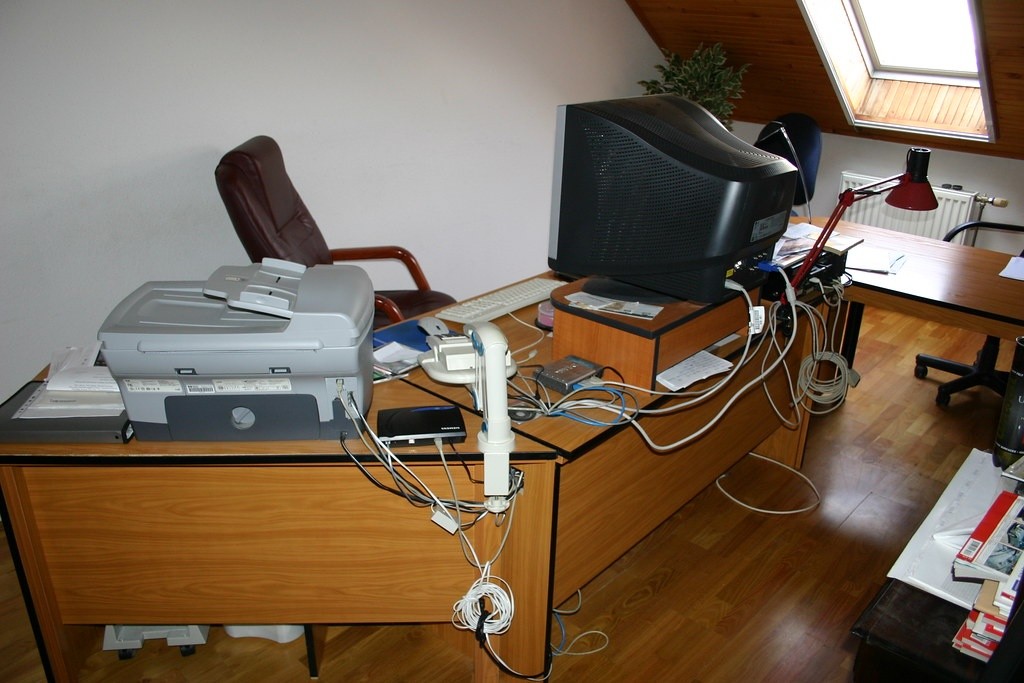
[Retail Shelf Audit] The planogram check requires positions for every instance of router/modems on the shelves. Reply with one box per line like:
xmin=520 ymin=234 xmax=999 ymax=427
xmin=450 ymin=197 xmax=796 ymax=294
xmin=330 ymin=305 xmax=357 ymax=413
xmin=377 ymin=405 xmax=467 ymax=448
xmin=531 ymin=354 xmax=605 ymax=395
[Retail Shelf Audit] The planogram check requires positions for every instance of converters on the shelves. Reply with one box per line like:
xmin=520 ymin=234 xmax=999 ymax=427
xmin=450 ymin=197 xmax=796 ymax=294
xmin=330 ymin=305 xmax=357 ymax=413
xmin=431 ymin=509 xmax=459 ymax=535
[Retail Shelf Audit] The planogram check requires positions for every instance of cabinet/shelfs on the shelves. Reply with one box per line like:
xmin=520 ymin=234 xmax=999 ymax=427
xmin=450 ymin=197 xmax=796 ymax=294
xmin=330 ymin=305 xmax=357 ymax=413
xmin=849 ymin=578 xmax=1024 ymax=683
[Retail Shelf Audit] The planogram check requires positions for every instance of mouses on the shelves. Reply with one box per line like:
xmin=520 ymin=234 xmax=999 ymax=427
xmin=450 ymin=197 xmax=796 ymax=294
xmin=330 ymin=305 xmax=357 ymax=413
xmin=417 ymin=316 xmax=449 ymax=336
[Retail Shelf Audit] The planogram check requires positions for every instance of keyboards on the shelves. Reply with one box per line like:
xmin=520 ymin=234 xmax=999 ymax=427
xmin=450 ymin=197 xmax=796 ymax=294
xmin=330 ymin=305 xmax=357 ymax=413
xmin=435 ymin=277 xmax=569 ymax=325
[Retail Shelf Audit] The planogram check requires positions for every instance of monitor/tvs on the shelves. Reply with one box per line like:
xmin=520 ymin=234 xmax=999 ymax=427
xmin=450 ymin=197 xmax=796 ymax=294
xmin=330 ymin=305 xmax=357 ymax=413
xmin=548 ymin=93 xmax=798 ymax=304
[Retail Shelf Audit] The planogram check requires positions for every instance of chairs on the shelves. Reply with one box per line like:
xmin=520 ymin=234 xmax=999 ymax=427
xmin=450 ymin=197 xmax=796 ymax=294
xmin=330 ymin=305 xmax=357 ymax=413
xmin=214 ymin=135 xmax=458 ymax=331
xmin=914 ymin=221 xmax=1024 ymax=407
xmin=755 ymin=113 xmax=823 ymax=219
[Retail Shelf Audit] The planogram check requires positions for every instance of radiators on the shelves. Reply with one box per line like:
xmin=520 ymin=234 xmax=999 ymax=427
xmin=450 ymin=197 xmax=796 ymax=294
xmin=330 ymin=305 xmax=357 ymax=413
xmin=836 ymin=171 xmax=1008 ymax=247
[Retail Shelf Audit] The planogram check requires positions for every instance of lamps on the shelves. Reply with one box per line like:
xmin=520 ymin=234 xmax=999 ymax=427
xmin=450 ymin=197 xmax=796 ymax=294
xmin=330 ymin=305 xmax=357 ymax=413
xmin=772 ymin=146 xmax=939 ymax=324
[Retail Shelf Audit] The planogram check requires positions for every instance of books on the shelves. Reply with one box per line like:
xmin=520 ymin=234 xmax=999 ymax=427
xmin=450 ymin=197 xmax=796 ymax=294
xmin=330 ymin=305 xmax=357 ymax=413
xmin=950 ymin=489 xmax=1024 ymax=663
xmin=770 ymin=221 xmax=909 ymax=275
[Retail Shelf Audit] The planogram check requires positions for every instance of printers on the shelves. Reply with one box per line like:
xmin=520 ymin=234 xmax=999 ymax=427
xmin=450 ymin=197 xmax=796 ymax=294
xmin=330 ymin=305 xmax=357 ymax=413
xmin=96 ymin=258 xmax=376 ymax=442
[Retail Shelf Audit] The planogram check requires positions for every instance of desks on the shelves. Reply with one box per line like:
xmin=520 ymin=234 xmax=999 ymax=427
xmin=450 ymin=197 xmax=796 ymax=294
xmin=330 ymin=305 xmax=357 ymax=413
xmin=304 ymin=270 xmax=830 ymax=680
xmin=0 ymin=362 xmax=558 ymax=683
xmin=795 ymin=217 xmax=1024 ymax=469
